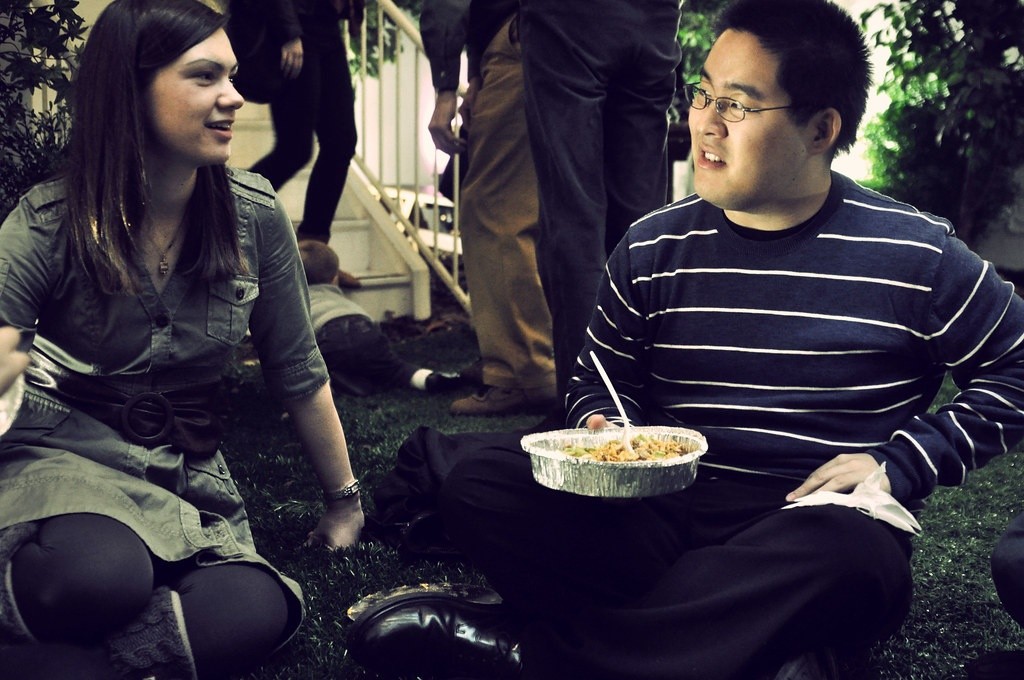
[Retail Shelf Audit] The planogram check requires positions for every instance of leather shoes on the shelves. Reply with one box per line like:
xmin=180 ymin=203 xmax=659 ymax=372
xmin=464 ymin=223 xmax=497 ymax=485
xmin=347 ymin=592 xmax=525 ymax=680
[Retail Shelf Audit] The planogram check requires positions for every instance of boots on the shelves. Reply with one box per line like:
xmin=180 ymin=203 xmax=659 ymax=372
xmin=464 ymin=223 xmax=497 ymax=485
xmin=0 ymin=591 xmax=197 ymax=680
xmin=0 ymin=522 xmax=42 ymax=645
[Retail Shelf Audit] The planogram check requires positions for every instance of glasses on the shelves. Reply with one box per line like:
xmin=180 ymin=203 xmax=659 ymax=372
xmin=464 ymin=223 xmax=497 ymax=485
xmin=683 ymin=82 xmax=791 ymax=123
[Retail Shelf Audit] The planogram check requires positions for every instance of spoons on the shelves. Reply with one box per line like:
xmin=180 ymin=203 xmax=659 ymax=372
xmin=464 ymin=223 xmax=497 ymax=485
xmin=590 ymin=350 xmax=638 ymax=455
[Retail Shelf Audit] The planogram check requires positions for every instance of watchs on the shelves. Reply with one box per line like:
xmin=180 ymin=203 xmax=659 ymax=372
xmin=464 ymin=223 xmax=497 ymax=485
xmin=324 ymin=478 xmax=361 ymax=500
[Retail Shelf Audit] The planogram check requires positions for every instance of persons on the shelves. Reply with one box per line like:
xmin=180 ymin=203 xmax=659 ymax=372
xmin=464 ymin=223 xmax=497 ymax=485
xmin=419 ymin=0 xmax=557 ymax=415
xmin=348 ymin=0 xmax=1024 ymax=680
xmin=0 ymin=0 xmax=364 ymax=680
xmin=296 ymin=240 xmax=479 ymax=394
xmin=519 ymin=0 xmax=683 ymax=403
xmin=230 ymin=0 xmax=359 ymax=241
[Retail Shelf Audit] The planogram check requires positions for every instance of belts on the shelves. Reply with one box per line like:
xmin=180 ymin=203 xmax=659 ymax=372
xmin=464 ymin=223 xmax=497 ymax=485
xmin=16 ymin=345 xmax=238 ymax=460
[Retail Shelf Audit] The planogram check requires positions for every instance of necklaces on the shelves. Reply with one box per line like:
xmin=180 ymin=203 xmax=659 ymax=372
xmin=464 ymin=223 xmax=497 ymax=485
xmin=148 ymin=210 xmax=185 ymax=275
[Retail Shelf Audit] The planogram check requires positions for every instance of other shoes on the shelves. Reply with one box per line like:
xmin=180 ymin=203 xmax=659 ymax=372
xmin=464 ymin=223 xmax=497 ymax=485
xmin=450 ymin=383 xmax=558 ymax=416
